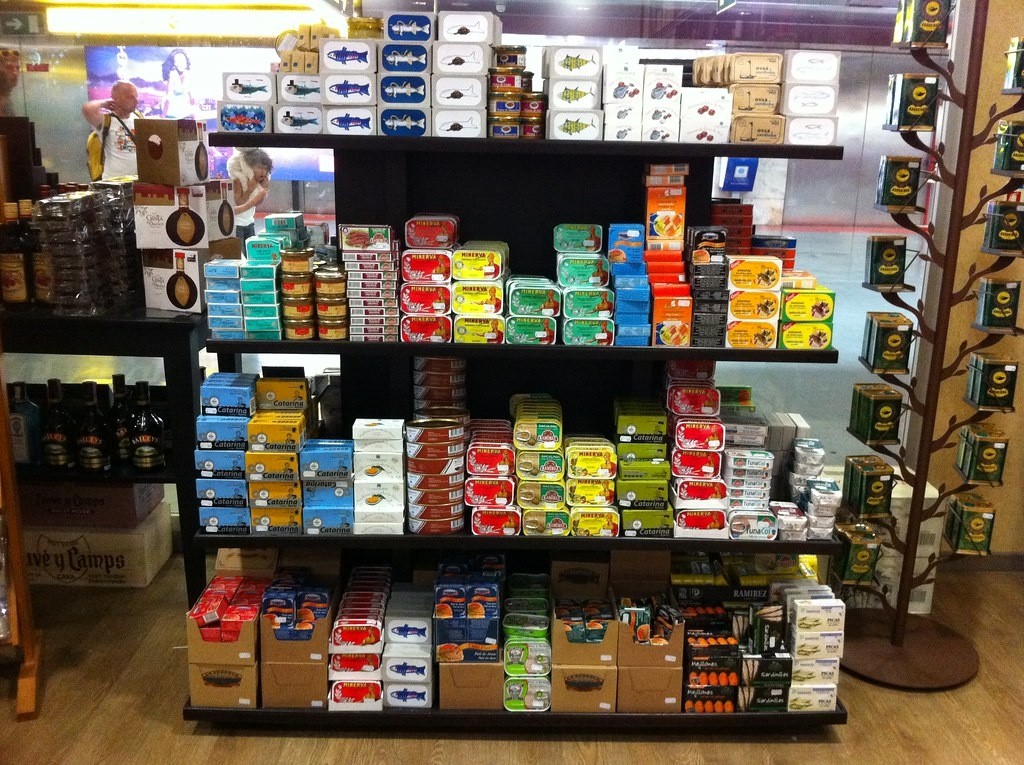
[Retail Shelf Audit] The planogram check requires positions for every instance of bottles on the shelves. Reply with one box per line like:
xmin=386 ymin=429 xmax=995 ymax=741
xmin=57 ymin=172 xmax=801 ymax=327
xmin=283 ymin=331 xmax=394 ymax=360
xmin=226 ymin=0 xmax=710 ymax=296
xmin=106 ymin=375 xmax=131 ymax=459
xmin=131 ymin=382 xmax=166 ymax=474
xmin=76 ymin=381 xmax=107 ymax=472
xmin=195 ymin=123 xmax=208 ymax=181
xmin=0 ymin=181 xmax=89 ymax=313
xmin=166 ymin=188 xmax=205 ymax=246
xmin=9 ymin=382 xmax=41 ymax=468
xmin=218 ymin=183 xmax=234 ymax=236
xmin=42 ymin=379 xmax=75 ymax=469
xmin=166 ymin=253 xmax=197 ymax=310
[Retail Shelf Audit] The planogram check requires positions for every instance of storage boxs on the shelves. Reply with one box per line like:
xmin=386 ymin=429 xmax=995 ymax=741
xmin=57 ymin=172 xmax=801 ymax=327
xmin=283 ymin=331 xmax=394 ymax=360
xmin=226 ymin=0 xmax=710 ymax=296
xmin=0 ymin=0 xmax=1024 ymax=714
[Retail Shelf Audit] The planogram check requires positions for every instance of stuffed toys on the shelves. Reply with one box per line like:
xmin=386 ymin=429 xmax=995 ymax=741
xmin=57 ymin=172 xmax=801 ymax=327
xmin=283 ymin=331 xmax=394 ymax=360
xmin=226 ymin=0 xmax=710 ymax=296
xmin=227 ymin=147 xmax=271 ymax=201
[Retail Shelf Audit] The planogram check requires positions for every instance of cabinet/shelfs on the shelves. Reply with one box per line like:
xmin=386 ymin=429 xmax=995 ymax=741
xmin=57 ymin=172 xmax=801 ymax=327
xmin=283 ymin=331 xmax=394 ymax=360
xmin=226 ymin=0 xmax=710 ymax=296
xmin=184 ymin=132 xmax=849 ymax=733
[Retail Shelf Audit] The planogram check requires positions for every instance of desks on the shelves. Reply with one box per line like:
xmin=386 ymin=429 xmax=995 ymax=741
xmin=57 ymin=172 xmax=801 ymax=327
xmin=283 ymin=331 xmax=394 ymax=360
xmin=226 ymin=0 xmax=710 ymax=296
xmin=0 ymin=305 xmax=211 ymax=607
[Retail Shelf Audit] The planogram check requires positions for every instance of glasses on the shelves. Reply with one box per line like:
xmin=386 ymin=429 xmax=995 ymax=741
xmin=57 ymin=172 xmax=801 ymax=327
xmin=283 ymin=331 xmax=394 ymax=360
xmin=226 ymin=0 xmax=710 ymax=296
xmin=0 ymin=49 xmax=21 ymax=61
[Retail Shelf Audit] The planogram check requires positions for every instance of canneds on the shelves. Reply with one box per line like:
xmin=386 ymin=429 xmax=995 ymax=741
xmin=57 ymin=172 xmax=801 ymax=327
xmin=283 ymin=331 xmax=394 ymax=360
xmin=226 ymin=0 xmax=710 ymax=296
xmin=834 ymin=0 xmax=1024 ymax=584
xmin=197 ymin=14 xmax=839 ymax=712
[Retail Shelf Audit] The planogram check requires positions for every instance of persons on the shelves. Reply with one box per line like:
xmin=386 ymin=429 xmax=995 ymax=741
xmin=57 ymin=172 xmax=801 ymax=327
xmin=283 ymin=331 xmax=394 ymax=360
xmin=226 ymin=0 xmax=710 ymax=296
xmin=79 ymin=80 xmax=142 ymax=179
xmin=159 ymin=48 xmax=201 ymax=118
xmin=234 ymin=150 xmax=271 ymax=258
xmin=584 ymin=321 xmax=613 ymax=346
xmin=580 ymin=260 xmax=608 ymax=285
xmin=584 ymin=291 xmax=613 ymax=316
xmin=574 ymin=226 xmax=601 ymax=251
xmin=469 ymin=253 xmax=500 ymax=278
xmin=527 ymin=319 xmax=554 ymax=344
xmin=532 ymin=291 xmax=560 ymax=316
xmin=0 ymin=48 xmax=21 ymax=116
xmin=470 ymin=287 xmax=501 ymax=313
xmin=472 ymin=320 xmax=503 ymax=343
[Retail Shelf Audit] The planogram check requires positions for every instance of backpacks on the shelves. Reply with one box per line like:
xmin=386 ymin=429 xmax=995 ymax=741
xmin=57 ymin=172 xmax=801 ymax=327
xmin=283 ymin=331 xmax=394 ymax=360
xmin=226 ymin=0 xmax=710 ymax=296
xmin=87 ymin=110 xmax=143 ymax=182
